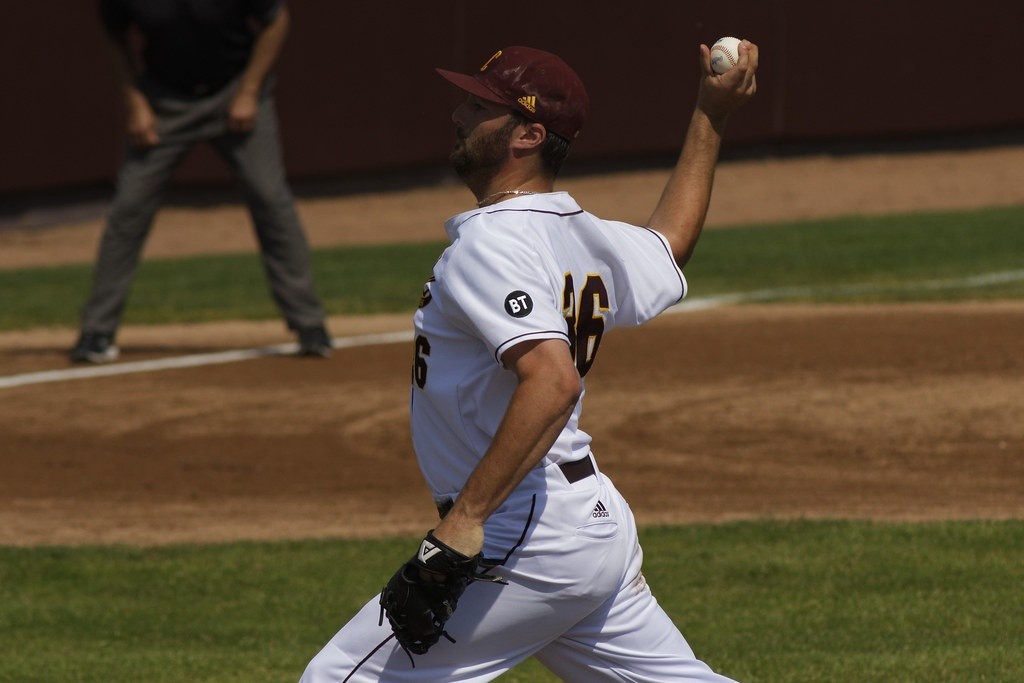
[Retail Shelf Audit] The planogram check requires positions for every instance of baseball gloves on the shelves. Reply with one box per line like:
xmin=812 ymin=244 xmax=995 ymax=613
xmin=378 ymin=528 xmax=486 ymax=654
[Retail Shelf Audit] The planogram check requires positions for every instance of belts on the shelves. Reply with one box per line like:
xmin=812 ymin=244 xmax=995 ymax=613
xmin=438 ymin=454 xmax=595 ymax=520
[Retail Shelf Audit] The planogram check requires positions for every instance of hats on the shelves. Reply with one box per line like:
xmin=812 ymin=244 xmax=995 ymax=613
xmin=436 ymin=45 xmax=590 ymax=146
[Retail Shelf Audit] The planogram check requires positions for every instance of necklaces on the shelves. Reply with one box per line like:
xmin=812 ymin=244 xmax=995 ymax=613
xmin=475 ymin=190 xmax=539 ymax=206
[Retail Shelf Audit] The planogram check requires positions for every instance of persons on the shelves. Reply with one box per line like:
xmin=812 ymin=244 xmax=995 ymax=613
xmin=69 ymin=0 xmax=335 ymax=366
xmin=295 ymin=36 xmax=761 ymax=683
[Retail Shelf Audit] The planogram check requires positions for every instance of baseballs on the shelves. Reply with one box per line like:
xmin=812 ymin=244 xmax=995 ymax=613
xmin=710 ymin=36 xmax=747 ymax=74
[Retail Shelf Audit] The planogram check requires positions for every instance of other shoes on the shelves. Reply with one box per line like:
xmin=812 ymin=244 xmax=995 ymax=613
xmin=298 ymin=328 xmax=332 ymax=359
xmin=70 ymin=332 xmax=119 ymax=363
